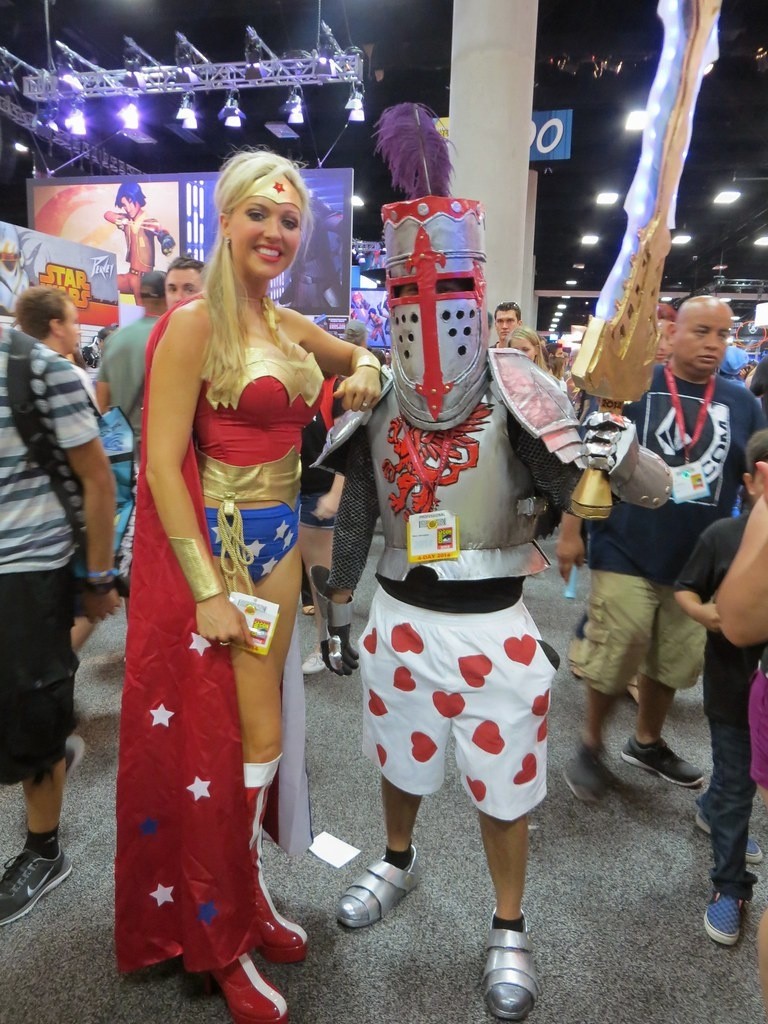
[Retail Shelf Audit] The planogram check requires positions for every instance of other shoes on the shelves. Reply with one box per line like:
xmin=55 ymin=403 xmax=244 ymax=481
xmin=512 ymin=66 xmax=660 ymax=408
xmin=703 ymin=891 xmax=742 ymax=946
xmin=696 ymin=810 xmax=762 ymax=863
xmin=481 ymin=908 xmax=539 ymax=1018
xmin=336 ymin=844 xmax=422 ymax=927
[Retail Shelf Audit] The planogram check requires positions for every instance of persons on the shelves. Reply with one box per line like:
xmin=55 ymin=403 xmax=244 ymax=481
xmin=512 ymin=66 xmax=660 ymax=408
xmin=717 ymin=462 xmax=768 ymax=1013
xmin=491 ymin=301 xmax=598 ymax=437
xmin=556 ymin=296 xmax=768 ymax=799
xmin=654 ymin=302 xmax=768 ymax=422
xmin=671 ymin=432 xmax=768 ymax=946
xmin=0 ymin=326 xmax=122 ymax=928
xmin=14 ymin=257 xmax=204 ymax=651
xmin=114 ymin=153 xmax=381 ymax=1024
xmin=301 ymin=319 xmax=391 ymax=674
xmin=306 ymin=280 xmax=590 ymax=1020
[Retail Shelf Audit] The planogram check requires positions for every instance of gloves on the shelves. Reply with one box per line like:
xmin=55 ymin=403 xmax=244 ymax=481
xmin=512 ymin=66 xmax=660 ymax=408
xmin=580 ymin=409 xmax=673 ymax=510
xmin=308 ymin=563 xmax=360 ymax=677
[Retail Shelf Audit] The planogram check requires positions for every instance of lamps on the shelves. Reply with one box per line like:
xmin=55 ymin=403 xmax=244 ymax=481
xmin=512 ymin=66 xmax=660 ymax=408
xmin=0 ymin=30 xmax=366 ymax=134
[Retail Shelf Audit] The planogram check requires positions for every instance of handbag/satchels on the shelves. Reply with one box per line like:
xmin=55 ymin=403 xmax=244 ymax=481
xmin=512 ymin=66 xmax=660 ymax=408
xmin=9 ymin=334 xmax=138 ymax=597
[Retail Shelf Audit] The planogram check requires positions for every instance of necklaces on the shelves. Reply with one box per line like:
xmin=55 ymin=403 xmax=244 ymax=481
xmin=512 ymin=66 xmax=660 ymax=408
xmin=242 ymin=295 xmax=280 ymax=345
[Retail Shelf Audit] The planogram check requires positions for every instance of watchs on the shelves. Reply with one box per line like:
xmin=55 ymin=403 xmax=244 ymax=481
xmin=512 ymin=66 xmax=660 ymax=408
xmin=88 ymin=581 xmax=114 ymax=595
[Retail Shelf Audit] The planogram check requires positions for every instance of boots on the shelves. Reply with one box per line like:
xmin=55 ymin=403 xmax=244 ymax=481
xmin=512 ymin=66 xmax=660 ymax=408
xmin=242 ymin=751 xmax=309 ymax=962
xmin=209 ymin=950 xmax=290 ymax=1024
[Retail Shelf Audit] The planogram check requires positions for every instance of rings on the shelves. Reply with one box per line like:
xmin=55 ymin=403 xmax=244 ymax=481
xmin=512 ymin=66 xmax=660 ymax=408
xmin=220 ymin=642 xmax=229 ymax=646
xmin=362 ymin=403 xmax=367 ymax=406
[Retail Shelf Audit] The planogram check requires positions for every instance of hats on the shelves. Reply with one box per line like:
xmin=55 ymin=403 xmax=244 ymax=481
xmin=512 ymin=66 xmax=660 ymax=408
xmin=140 ymin=270 xmax=166 ymax=299
xmin=720 ymin=346 xmax=750 ymax=375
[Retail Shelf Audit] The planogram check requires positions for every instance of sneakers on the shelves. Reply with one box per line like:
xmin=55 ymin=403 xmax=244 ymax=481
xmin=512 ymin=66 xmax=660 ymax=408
xmin=622 ymin=735 xmax=705 ymax=786
xmin=562 ymin=743 xmax=612 ymax=804
xmin=302 ymin=653 xmax=326 ymax=674
xmin=0 ymin=842 xmax=74 ymax=926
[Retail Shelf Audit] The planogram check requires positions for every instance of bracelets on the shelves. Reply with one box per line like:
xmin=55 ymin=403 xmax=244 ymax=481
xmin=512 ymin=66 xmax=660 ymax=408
xmin=355 ymin=355 xmax=380 ymax=371
xmin=168 ymin=537 xmax=223 ymax=603
xmin=88 ymin=568 xmax=119 ymax=578
